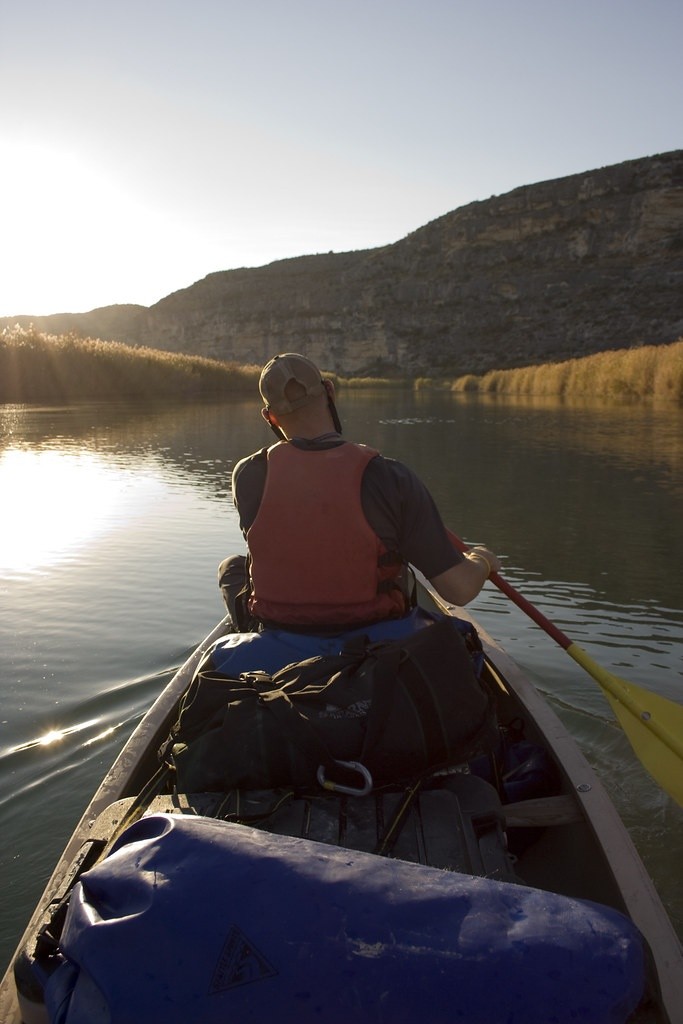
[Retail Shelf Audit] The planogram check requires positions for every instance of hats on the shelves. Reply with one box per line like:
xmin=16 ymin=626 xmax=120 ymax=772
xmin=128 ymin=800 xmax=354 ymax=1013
xmin=259 ymin=353 xmax=324 ymax=417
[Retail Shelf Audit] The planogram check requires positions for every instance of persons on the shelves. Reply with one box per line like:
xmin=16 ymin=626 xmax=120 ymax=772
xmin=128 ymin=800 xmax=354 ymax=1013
xmin=218 ymin=352 xmax=502 ymax=634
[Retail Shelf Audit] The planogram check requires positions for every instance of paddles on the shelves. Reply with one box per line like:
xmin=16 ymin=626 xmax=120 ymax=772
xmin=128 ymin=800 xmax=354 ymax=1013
xmin=444 ymin=527 xmax=683 ymax=807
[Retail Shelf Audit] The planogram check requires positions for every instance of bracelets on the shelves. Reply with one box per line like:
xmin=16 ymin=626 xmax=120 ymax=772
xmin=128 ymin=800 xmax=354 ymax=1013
xmin=468 ymin=552 xmax=491 ymax=578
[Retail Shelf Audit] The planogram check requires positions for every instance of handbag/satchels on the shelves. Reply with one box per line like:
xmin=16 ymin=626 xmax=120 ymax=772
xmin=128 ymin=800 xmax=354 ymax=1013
xmin=158 ymin=621 xmax=489 ymax=791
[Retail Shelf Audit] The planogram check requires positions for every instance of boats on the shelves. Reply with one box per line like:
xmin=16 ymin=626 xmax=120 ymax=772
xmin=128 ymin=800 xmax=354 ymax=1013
xmin=2 ymin=565 xmax=681 ymax=1024
